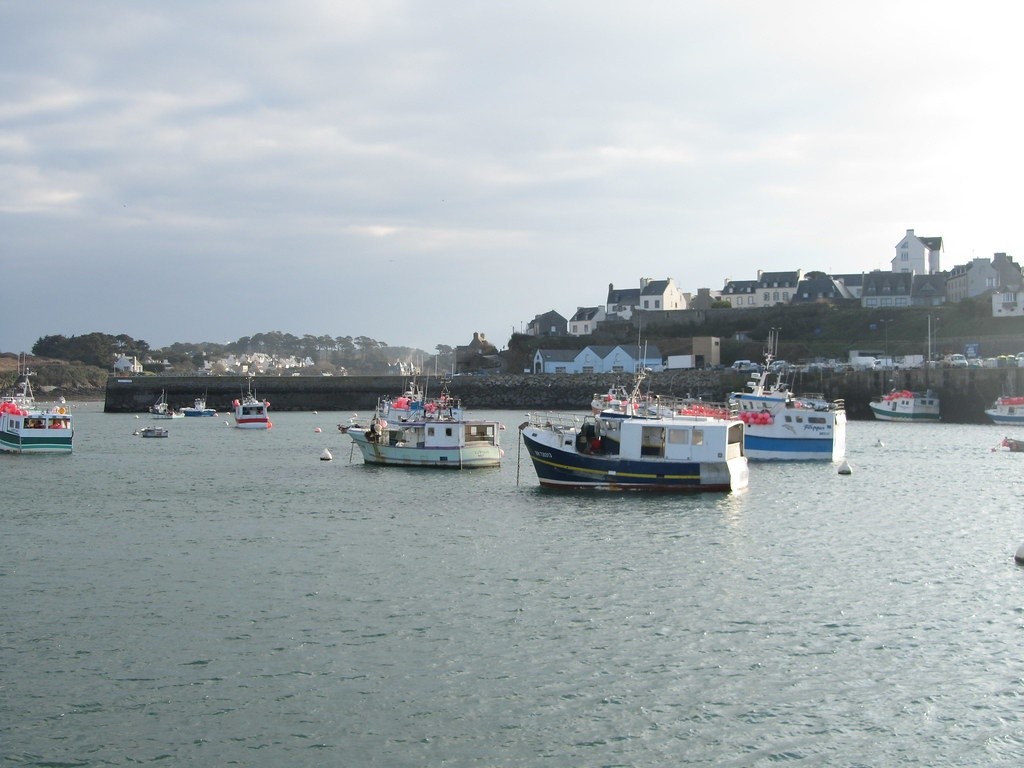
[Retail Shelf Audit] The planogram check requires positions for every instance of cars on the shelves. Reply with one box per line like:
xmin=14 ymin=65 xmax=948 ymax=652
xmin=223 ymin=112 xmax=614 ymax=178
xmin=731 ymin=360 xmax=750 ymax=369
xmin=950 ymin=354 xmax=968 ymax=367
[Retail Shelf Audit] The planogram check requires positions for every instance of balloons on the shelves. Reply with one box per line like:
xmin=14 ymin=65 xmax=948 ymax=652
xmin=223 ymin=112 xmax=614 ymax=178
xmin=737 ymin=412 xmax=774 ymax=425
xmin=392 ymin=397 xmax=410 ymax=410
xmin=235 ymin=399 xmax=240 ymax=406
xmin=0 ymin=402 xmax=29 ymax=417
xmin=265 ymin=402 xmax=270 ymax=406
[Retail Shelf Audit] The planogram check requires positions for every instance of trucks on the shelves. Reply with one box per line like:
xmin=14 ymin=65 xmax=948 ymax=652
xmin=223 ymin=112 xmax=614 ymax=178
xmin=895 ymin=355 xmax=924 ymax=370
xmin=663 ymin=354 xmax=705 ymax=371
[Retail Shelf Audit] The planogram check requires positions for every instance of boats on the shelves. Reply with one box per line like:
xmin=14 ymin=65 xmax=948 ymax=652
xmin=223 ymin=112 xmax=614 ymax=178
xmin=140 ymin=426 xmax=168 ymax=438
xmin=519 ymin=340 xmax=749 ymax=493
xmin=868 ymin=388 xmax=940 ymax=423
xmin=180 ymin=389 xmax=216 ymax=416
xmin=148 ymin=388 xmax=185 ymax=418
xmin=347 ymin=355 xmax=501 ymax=470
xmin=1002 ymin=437 xmax=1024 ymax=453
xmin=984 ymin=384 xmax=1024 ymax=426
xmin=0 ymin=354 xmax=75 ymax=454
xmin=590 ymin=330 xmax=846 ymax=462
xmin=234 ymin=380 xmax=269 ymax=428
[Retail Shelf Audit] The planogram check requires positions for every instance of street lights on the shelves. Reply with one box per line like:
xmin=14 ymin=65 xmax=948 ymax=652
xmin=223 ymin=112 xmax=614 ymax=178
xmin=880 ymin=319 xmax=893 ymax=370
xmin=932 ymin=317 xmax=939 ymax=362
